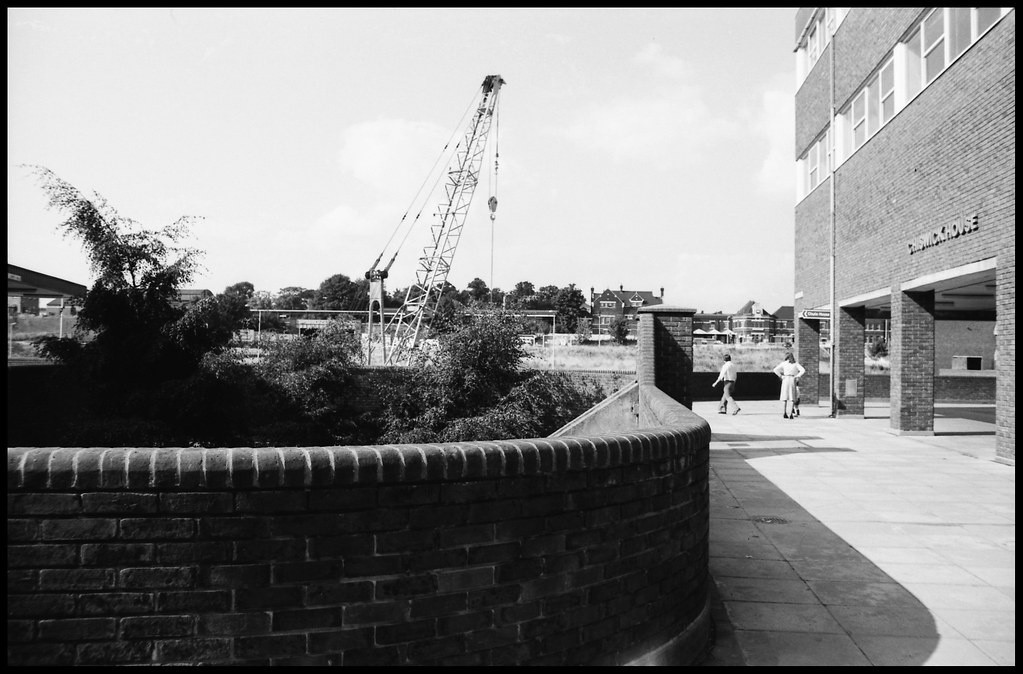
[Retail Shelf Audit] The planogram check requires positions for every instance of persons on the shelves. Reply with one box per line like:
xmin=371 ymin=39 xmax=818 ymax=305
xmin=773 ymin=352 xmax=806 ymax=419
xmin=793 ymin=380 xmax=800 ymax=417
xmin=712 ymin=354 xmax=741 ymax=415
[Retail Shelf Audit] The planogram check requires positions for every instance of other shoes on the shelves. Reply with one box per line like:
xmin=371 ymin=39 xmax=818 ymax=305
xmin=784 ymin=413 xmax=789 ymax=418
xmin=718 ymin=410 xmax=726 ymax=414
xmin=733 ymin=408 xmax=740 ymax=415
xmin=790 ymin=414 xmax=793 ymax=419
